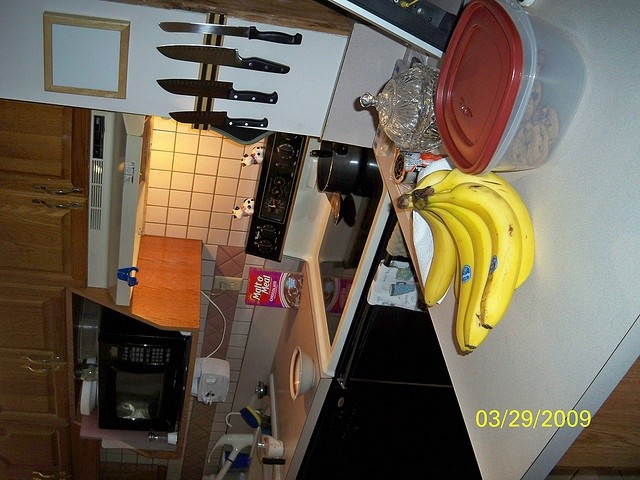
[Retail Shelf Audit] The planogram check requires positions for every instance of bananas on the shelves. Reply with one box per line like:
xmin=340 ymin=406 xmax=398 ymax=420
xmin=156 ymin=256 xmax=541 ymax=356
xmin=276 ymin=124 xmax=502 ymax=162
xmin=391 ymin=170 xmax=535 ymax=357
